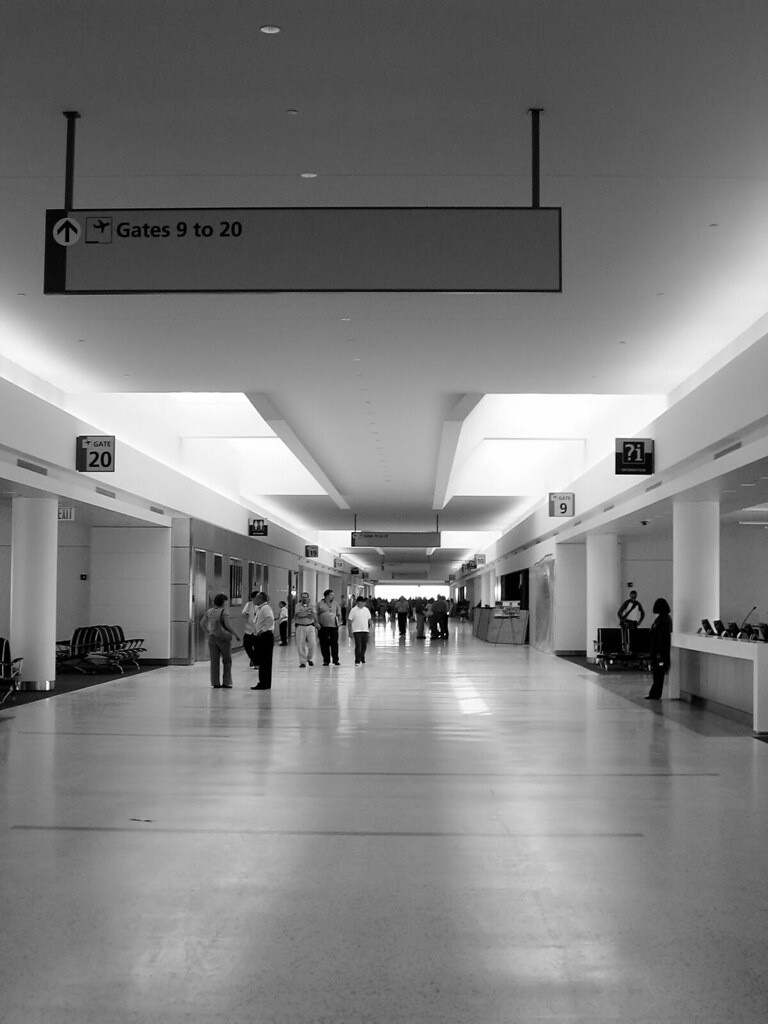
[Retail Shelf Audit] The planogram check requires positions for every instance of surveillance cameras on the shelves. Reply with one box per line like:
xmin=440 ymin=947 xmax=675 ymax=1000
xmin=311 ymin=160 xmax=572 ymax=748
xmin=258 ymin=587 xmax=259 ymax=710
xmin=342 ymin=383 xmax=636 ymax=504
xmin=642 ymin=521 xmax=648 ymax=525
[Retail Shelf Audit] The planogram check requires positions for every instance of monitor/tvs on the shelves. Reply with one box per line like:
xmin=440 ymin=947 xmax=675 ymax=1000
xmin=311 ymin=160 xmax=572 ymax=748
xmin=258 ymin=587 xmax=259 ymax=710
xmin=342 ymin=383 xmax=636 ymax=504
xmin=701 ymin=619 xmax=768 ymax=644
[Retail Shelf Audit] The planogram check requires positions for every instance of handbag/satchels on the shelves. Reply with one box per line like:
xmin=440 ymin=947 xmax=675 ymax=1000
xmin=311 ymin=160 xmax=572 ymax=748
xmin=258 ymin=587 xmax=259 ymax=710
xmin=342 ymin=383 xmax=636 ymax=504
xmin=214 ymin=610 xmax=233 ymax=642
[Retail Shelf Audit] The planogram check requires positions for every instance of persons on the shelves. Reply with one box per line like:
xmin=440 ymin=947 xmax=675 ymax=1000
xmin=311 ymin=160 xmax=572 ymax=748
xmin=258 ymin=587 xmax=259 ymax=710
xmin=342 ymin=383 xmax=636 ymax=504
xmin=617 ymin=590 xmax=645 ymax=655
xmin=242 ymin=590 xmax=455 ymax=690
xmin=200 ymin=594 xmax=240 ymax=688
xmin=644 ymin=598 xmax=673 ymax=700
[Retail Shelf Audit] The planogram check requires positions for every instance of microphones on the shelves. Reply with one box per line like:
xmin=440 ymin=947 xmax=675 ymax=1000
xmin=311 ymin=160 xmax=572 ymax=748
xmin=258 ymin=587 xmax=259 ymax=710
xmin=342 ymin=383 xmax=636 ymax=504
xmin=741 ymin=606 xmax=757 ymax=631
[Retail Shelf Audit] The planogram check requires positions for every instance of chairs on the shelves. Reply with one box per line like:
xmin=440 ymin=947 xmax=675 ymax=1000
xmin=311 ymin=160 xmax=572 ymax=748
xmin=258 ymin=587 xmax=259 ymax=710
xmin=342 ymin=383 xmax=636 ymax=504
xmin=0 ymin=638 xmax=23 ymax=705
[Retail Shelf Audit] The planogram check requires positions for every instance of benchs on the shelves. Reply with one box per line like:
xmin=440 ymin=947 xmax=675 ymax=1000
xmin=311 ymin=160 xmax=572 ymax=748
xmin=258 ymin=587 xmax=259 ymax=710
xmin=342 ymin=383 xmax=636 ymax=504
xmin=56 ymin=625 xmax=145 ymax=676
xmin=593 ymin=628 xmax=652 ymax=672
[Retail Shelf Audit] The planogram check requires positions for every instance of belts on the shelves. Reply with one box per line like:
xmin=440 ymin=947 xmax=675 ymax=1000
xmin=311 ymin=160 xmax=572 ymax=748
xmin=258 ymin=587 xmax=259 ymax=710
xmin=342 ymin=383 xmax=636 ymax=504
xmin=298 ymin=624 xmax=313 ymax=626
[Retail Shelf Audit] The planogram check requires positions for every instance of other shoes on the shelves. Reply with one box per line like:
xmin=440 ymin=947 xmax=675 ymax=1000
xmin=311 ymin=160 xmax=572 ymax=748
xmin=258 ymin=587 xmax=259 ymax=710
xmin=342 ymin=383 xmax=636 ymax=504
xmin=214 ymin=685 xmax=221 ymax=688
xmin=300 ymin=664 xmax=305 ymax=667
xmin=254 ymin=665 xmax=259 ymax=669
xmin=251 ymin=684 xmax=267 ymax=690
xmin=355 ymin=660 xmax=360 ymax=664
xmin=279 ymin=643 xmax=287 ymax=646
xmin=307 ymin=660 xmax=313 ymax=666
xmin=417 ymin=635 xmax=426 ymax=639
xmin=221 ymin=684 xmax=232 ymax=688
xmin=361 ymin=659 xmax=365 ymax=663
xmin=323 ymin=662 xmax=329 ymax=666
xmin=250 ymin=659 xmax=254 ymax=667
xmin=333 ymin=661 xmax=340 ymax=665
